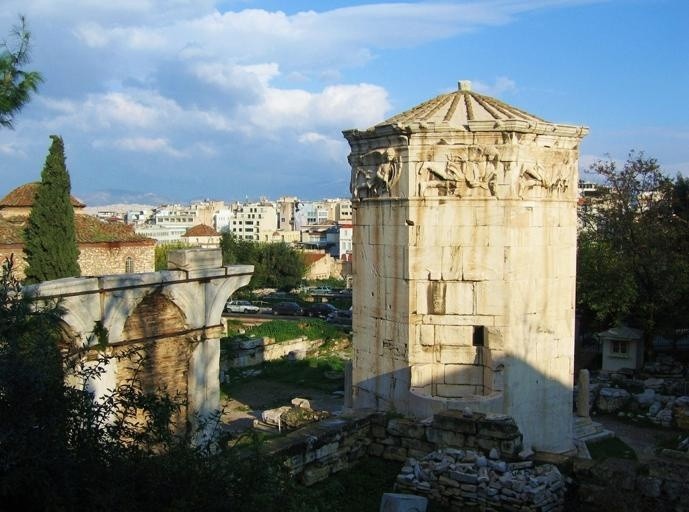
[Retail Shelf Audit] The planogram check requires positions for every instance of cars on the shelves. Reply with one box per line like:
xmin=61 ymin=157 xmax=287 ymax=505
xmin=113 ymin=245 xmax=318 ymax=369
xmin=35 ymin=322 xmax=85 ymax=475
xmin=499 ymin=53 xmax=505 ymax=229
xmin=227 ymin=300 xmax=260 ymax=314
xmin=327 ymin=311 xmax=352 ymax=324
xmin=251 ymin=301 xmax=272 ymax=314
xmin=303 ymin=303 xmax=339 ymax=317
xmin=272 ymin=301 xmax=304 ymax=316
xmin=339 ymin=287 xmax=353 ymax=295
xmin=263 ymin=291 xmax=303 ymax=305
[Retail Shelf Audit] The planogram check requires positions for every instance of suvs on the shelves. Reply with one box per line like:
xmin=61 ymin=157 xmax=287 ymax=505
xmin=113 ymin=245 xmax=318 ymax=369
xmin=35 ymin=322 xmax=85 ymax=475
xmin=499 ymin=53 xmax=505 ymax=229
xmin=310 ymin=286 xmax=332 ymax=295
